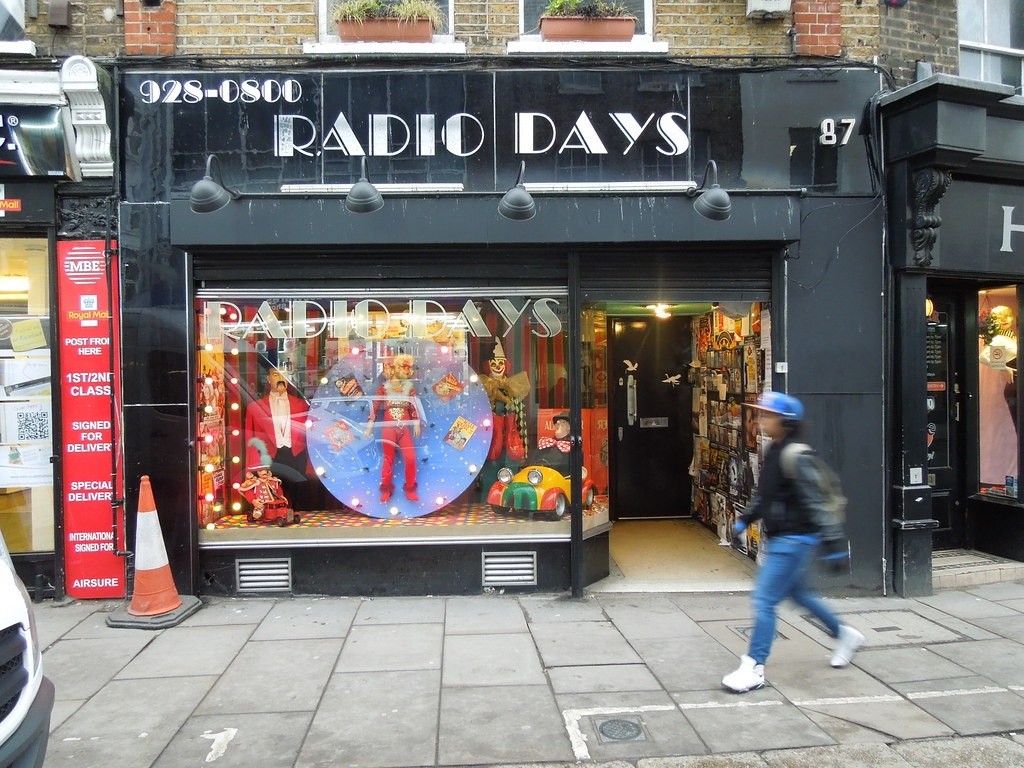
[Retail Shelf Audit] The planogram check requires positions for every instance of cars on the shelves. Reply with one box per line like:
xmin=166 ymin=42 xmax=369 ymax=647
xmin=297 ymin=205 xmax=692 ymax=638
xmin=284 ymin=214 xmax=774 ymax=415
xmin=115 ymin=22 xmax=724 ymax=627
xmin=246 ymin=501 xmax=301 ymax=526
xmin=486 ymin=459 xmax=600 ymax=521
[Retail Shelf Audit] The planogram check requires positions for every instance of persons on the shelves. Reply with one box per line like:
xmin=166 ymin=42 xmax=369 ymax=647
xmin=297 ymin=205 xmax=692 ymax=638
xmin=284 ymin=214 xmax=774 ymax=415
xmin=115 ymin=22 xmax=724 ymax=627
xmin=244 ymin=349 xmax=308 ymax=511
xmin=365 ymin=356 xmax=425 ymax=503
xmin=722 ymin=393 xmax=864 ymax=692
xmin=238 ymin=439 xmax=288 ymax=518
xmin=468 ymin=336 xmax=530 ymax=504
xmin=525 ymin=411 xmax=582 ymax=478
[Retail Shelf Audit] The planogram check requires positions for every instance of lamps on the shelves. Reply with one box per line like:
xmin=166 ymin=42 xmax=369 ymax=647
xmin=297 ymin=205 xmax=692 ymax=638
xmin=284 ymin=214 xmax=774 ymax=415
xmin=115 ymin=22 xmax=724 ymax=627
xmin=189 ymin=154 xmax=241 ymax=214
xmin=498 ymin=160 xmax=536 ymax=221
xmin=686 ymin=159 xmax=732 ymax=222
xmin=344 ymin=155 xmax=385 ymax=215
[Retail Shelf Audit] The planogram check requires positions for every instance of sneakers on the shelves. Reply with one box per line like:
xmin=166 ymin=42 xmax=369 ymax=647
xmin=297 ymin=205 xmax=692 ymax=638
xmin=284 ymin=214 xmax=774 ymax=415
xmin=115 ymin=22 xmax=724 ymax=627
xmin=722 ymin=653 xmax=764 ymax=692
xmin=829 ymin=625 xmax=866 ymax=668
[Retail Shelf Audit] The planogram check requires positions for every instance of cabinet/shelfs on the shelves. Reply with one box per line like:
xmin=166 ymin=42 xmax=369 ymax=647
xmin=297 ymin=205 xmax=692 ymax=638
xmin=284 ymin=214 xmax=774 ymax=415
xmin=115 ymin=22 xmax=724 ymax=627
xmin=689 ymin=347 xmax=747 ymax=550
xmin=343 ymin=312 xmax=472 ymax=410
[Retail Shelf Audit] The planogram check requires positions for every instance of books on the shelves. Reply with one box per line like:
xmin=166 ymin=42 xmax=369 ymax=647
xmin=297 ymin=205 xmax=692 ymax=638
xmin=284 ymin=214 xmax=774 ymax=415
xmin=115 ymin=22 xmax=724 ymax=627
xmin=688 ymin=303 xmax=761 ymax=561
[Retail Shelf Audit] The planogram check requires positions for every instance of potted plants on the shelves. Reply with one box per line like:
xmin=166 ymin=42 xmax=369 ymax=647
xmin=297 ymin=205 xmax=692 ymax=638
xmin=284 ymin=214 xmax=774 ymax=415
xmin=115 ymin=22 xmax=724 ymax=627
xmin=331 ymin=0 xmax=445 ymax=43
xmin=539 ymin=0 xmax=638 ymax=42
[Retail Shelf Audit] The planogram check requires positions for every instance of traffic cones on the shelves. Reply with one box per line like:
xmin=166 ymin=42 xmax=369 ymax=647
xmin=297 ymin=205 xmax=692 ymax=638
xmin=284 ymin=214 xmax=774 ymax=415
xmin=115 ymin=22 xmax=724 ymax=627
xmin=105 ymin=473 xmax=204 ymax=632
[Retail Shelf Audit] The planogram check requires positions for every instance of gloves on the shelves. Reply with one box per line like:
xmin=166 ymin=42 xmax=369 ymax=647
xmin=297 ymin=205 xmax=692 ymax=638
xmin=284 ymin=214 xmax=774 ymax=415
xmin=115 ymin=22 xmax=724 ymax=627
xmin=731 ymin=521 xmax=747 ymax=551
xmin=822 ymin=541 xmax=848 ymax=574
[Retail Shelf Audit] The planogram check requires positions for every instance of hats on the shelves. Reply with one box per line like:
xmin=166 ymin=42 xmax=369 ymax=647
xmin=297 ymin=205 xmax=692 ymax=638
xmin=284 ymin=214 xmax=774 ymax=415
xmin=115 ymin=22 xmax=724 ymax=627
xmin=740 ymin=391 xmax=804 ymax=420
